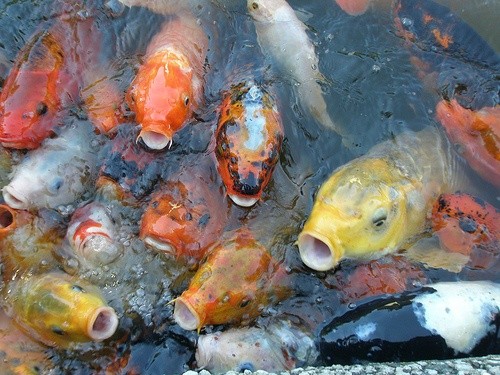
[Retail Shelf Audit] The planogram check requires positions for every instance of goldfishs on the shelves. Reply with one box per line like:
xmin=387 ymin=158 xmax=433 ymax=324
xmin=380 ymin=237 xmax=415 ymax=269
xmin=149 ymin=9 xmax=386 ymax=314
xmin=0 ymin=0 xmax=500 ymax=375
